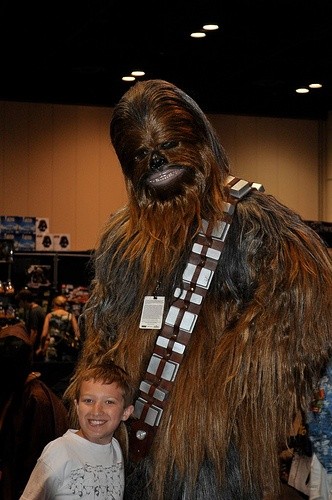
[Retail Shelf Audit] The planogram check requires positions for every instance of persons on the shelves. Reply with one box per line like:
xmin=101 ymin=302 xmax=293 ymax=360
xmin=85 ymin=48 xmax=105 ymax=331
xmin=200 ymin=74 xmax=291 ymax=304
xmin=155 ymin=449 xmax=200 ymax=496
xmin=20 ymin=364 xmax=134 ymax=496
xmin=11 ymin=288 xmax=81 ymax=359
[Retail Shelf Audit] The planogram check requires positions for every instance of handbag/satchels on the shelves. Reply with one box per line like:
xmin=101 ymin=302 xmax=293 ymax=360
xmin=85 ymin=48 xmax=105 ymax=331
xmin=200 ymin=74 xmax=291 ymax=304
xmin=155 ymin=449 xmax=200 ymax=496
xmin=47 ymin=330 xmax=83 ymax=357
